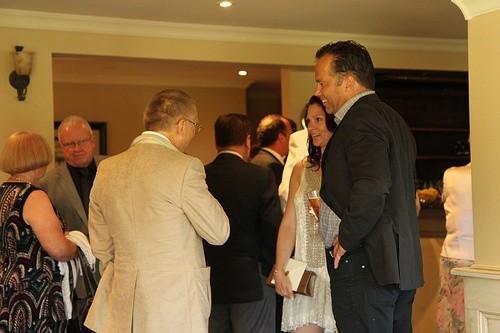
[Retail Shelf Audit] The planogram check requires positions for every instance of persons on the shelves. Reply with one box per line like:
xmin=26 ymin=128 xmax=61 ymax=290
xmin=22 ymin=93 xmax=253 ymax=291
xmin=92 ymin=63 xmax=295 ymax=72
xmin=0 ymin=132 xmax=78 ymax=333
xmin=435 ymin=162 xmax=473 ymax=333
xmin=249 ymin=113 xmax=298 ymax=188
xmin=267 ymin=94 xmax=339 ymax=332
xmin=314 ymin=40 xmax=424 ymax=332
xmin=84 ymin=88 xmax=230 ymax=332
xmin=32 ymin=114 xmax=112 ymax=246
xmin=202 ymin=114 xmax=283 ymax=333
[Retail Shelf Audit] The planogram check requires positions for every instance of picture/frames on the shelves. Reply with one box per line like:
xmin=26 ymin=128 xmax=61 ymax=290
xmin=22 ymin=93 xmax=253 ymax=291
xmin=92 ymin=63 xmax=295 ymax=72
xmin=54 ymin=121 xmax=107 ymax=161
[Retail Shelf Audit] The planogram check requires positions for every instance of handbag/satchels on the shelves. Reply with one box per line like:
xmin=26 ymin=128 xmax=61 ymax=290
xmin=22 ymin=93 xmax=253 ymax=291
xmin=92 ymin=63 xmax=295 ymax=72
xmin=266 ymin=264 xmax=319 ymax=297
xmin=78 ymin=298 xmax=93 ymax=333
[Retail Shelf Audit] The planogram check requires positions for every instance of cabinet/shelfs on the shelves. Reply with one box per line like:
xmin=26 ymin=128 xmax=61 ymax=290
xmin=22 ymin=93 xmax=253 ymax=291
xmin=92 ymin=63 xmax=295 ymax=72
xmin=375 ymin=70 xmax=469 ymax=180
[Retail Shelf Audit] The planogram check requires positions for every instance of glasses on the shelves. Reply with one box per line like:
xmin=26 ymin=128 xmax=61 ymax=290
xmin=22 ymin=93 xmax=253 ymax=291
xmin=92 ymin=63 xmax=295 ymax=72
xmin=185 ymin=118 xmax=202 ymax=135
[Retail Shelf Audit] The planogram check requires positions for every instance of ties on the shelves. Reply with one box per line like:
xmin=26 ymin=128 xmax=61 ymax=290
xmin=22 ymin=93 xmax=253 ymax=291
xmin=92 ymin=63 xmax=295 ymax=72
xmin=80 ymin=168 xmax=91 ymax=217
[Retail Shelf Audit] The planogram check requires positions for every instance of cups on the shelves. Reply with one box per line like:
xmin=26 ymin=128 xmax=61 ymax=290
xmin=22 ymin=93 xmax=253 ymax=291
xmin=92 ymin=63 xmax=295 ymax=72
xmin=306 ymin=189 xmax=324 ymax=221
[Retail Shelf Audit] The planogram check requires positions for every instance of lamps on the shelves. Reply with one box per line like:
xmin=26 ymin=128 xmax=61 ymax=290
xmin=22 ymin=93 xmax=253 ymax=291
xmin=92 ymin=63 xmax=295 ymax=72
xmin=9 ymin=46 xmax=35 ymax=101
